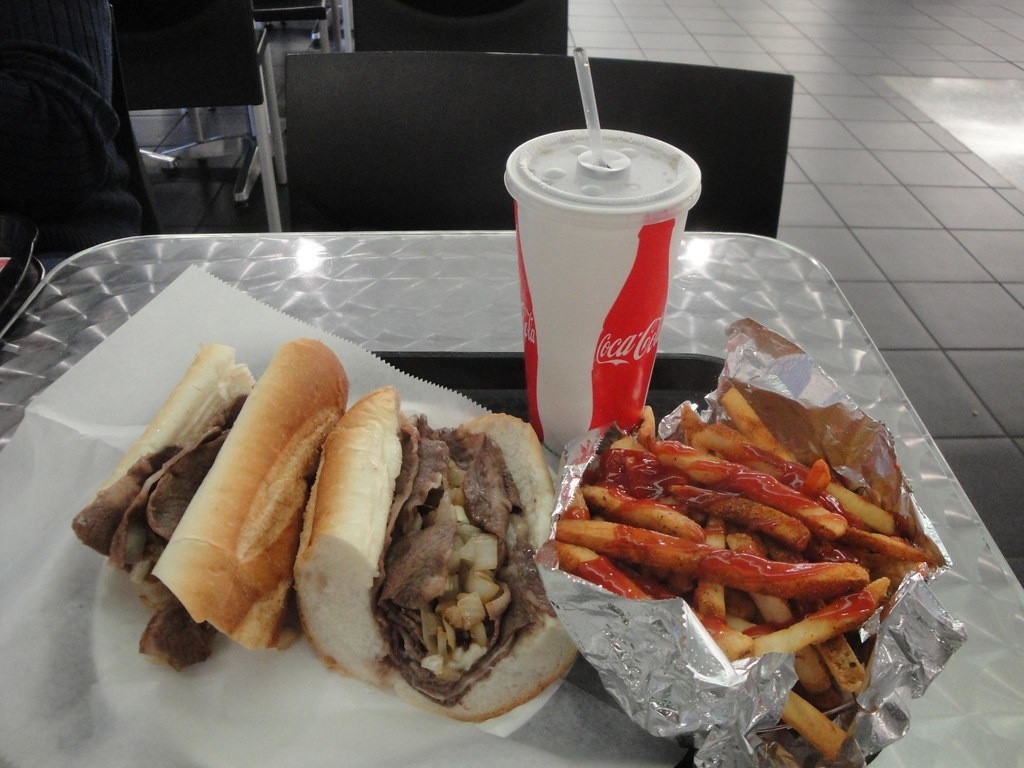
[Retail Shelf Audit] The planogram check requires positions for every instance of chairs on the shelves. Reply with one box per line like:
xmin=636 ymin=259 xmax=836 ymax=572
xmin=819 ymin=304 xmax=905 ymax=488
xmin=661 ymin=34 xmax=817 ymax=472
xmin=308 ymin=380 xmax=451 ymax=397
xmin=106 ymin=0 xmax=795 ymax=243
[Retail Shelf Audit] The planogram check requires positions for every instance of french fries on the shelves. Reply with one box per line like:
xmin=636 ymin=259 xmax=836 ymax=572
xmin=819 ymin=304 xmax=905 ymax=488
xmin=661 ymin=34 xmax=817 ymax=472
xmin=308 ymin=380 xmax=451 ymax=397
xmin=549 ymin=385 xmax=935 ymax=765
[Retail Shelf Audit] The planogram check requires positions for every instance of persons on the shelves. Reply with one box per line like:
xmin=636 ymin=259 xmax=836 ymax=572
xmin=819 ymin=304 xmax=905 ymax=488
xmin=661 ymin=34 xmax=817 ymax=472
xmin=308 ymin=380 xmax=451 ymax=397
xmin=1 ymin=0 xmax=143 ymax=277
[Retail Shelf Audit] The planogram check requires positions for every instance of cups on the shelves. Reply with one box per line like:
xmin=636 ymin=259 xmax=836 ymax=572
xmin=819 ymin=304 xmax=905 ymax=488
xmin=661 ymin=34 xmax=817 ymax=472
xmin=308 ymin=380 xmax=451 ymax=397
xmin=502 ymin=129 xmax=703 ymax=457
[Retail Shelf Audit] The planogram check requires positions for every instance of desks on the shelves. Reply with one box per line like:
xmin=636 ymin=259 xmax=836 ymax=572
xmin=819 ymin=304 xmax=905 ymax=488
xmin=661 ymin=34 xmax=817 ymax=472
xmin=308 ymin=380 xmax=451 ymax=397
xmin=0 ymin=229 xmax=1024 ymax=768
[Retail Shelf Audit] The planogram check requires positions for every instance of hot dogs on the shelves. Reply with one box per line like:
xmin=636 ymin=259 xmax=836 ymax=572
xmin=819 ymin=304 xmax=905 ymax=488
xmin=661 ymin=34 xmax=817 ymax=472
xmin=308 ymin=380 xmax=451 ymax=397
xmin=68 ymin=335 xmax=355 ymax=672
xmin=289 ymin=385 xmax=581 ymax=726
